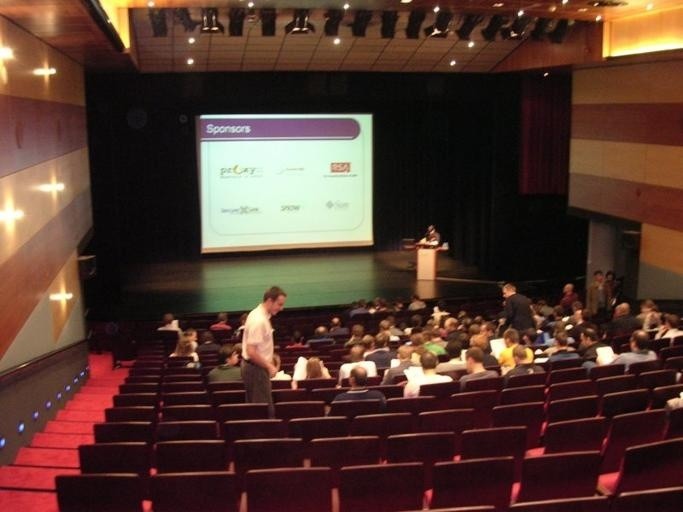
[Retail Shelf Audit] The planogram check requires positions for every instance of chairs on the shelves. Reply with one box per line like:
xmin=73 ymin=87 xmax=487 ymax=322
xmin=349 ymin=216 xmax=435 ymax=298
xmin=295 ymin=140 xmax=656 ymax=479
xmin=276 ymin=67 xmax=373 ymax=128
xmin=55 ymin=299 xmax=683 ymax=512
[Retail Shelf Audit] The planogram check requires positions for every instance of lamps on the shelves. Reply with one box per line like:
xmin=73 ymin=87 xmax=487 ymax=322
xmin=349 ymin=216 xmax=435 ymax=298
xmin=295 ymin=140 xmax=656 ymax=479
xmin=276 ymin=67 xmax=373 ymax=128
xmin=148 ymin=6 xmax=569 ymax=44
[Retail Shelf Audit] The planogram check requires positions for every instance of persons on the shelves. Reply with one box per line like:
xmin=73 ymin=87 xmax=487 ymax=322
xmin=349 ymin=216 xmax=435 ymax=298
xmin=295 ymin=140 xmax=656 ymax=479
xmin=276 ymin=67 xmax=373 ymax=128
xmin=159 ymin=271 xmax=683 ymax=418
xmin=419 ymin=225 xmax=441 ymax=248
